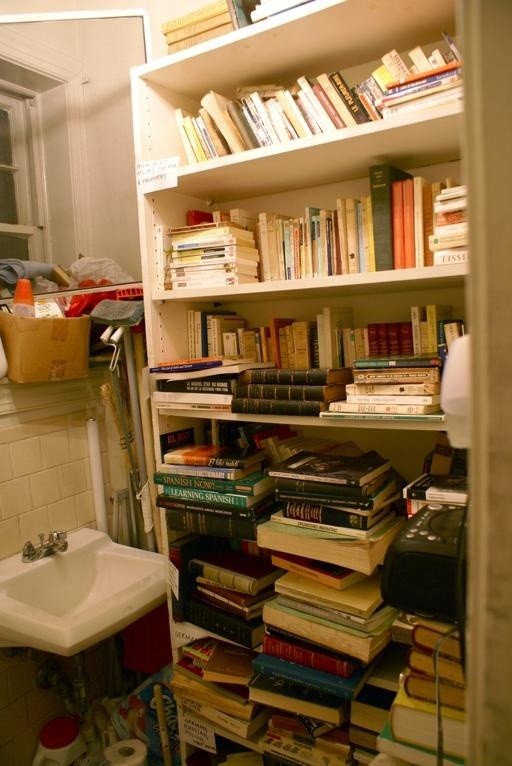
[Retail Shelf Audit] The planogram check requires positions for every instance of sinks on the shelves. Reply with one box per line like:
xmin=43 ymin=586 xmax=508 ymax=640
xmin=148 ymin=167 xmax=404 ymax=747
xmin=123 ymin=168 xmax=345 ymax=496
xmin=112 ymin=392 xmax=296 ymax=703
xmin=0 ymin=553 xmax=155 ymax=620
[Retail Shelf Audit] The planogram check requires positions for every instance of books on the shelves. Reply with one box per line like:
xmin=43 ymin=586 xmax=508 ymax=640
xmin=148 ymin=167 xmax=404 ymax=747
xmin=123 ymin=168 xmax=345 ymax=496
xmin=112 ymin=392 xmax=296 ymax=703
xmin=160 ymin=1 xmax=309 ymax=57
xmin=152 ymin=303 xmax=467 ymax=421
xmin=167 ymin=164 xmax=467 ymax=289
xmin=173 ymin=31 xmax=465 ymax=165
xmin=153 ymin=422 xmax=468 ymax=766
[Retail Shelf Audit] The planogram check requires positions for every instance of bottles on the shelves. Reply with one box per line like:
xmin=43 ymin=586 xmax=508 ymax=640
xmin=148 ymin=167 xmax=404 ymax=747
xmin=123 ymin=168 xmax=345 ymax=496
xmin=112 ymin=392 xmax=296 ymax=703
xmin=11 ymin=278 xmax=36 ymax=320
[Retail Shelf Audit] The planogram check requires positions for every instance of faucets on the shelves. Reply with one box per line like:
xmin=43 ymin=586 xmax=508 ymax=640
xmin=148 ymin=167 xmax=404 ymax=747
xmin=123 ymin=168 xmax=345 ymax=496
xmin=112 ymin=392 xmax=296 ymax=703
xmin=35 ymin=531 xmax=68 ymax=559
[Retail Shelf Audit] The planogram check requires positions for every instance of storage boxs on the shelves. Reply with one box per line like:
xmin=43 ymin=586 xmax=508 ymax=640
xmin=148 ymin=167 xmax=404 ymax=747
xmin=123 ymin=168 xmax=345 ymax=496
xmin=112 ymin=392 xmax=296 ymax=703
xmin=1 ymin=310 xmax=92 ymax=385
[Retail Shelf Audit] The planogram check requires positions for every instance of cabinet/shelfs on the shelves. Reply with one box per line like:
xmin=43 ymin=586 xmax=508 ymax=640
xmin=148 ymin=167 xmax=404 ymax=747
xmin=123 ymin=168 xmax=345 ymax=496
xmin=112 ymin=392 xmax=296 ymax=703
xmin=129 ymin=0 xmax=512 ymax=766
